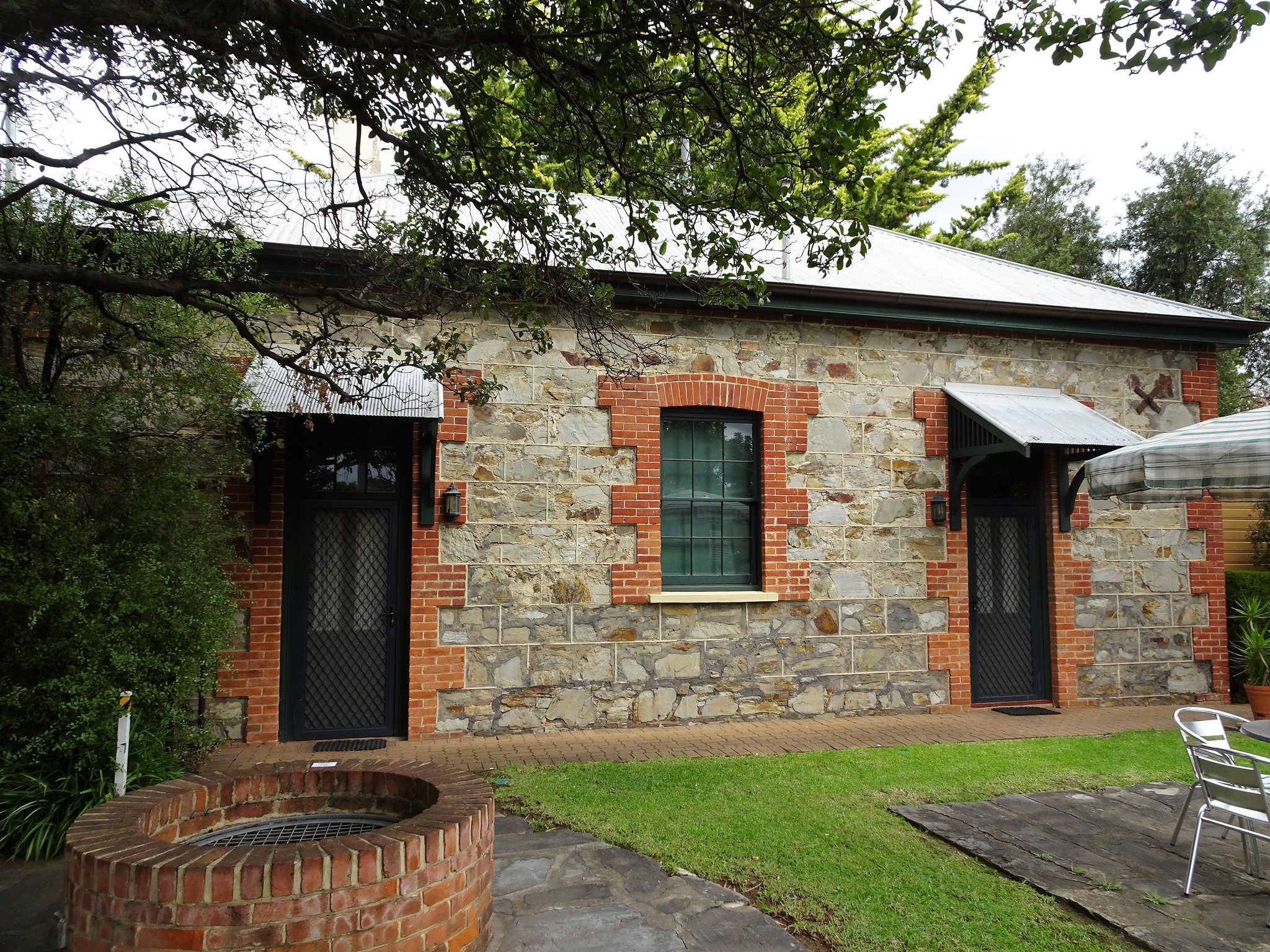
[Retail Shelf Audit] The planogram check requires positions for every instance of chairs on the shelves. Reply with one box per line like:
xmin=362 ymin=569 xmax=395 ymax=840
xmin=1169 ymin=706 xmax=1270 ymax=927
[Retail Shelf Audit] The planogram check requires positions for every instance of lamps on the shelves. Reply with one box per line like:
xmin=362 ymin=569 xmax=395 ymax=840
xmin=442 ymin=482 xmax=462 ymax=522
xmin=930 ymin=492 xmax=947 ymax=526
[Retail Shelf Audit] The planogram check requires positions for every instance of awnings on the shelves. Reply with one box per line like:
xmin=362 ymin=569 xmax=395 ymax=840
xmin=226 ymin=342 xmax=444 ymax=527
xmin=941 ymin=380 xmax=1146 ymax=529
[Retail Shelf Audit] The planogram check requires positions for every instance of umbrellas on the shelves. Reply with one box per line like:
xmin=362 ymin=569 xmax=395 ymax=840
xmin=661 ymin=490 xmax=749 ymax=576
xmin=1085 ymin=406 xmax=1270 ymax=503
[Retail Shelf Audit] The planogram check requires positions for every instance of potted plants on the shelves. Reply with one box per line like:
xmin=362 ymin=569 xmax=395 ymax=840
xmin=1226 ymin=596 xmax=1270 ymax=721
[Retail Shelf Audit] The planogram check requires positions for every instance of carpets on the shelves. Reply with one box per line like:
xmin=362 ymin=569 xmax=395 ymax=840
xmin=313 ymin=739 xmax=387 ymax=751
xmin=991 ymin=706 xmax=1062 ymax=716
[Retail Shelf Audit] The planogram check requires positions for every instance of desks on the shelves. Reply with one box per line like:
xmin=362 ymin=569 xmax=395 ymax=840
xmin=1240 ymin=719 xmax=1270 ymax=743
